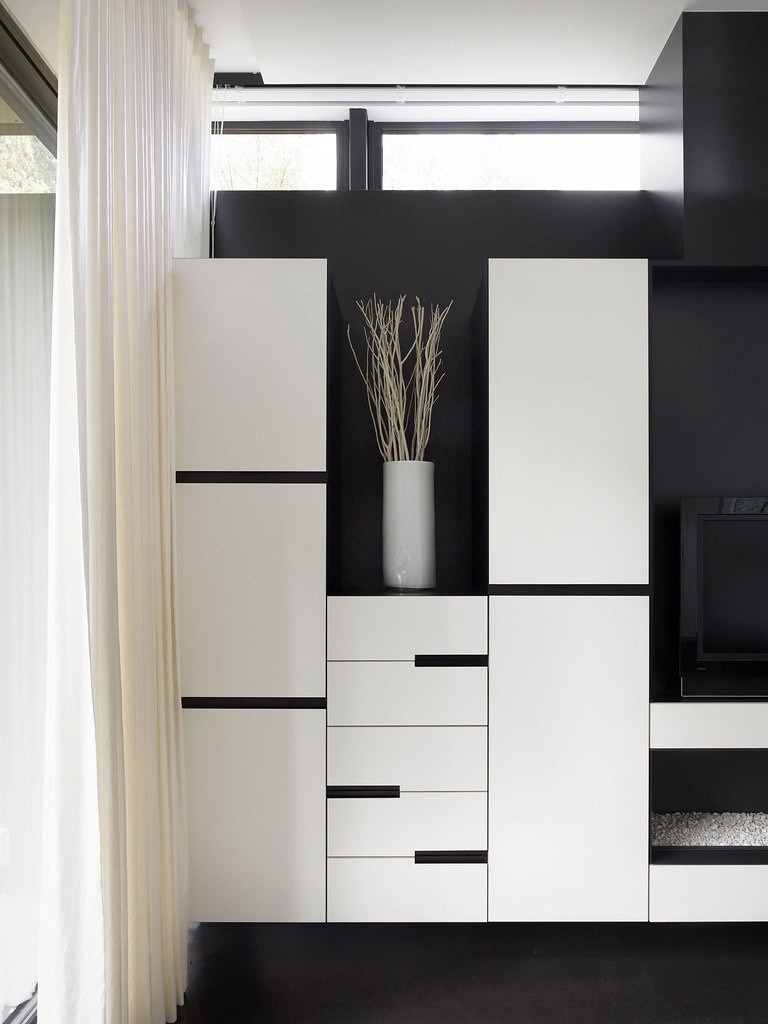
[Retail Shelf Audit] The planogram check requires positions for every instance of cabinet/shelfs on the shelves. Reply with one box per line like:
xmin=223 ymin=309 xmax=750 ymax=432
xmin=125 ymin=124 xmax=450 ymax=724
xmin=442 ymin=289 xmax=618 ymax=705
xmin=170 ymin=257 xmax=340 ymax=923
xmin=469 ymin=257 xmax=649 ymax=922
xmin=650 ymin=680 xmax=768 ymax=922
xmin=327 ymin=596 xmax=489 ymax=922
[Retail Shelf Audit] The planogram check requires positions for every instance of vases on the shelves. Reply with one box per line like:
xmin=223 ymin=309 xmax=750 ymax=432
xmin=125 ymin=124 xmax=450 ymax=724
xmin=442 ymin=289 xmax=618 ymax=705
xmin=384 ymin=461 xmax=438 ymax=589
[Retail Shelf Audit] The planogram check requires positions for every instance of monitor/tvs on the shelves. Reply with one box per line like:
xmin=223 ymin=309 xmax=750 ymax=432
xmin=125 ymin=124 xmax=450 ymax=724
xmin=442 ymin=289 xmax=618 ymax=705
xmin=680 ymin=497 xmax=768 ymax=697
